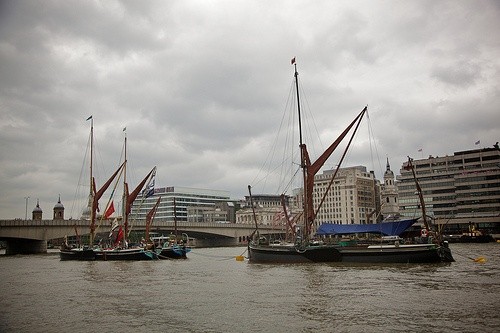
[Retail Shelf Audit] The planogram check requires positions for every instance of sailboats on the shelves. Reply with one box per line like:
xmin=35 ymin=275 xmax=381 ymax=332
xmin=246 ymin=56 xmax=455 ymax=264
xmin=59 ymin=114 xmax=189 ymax=261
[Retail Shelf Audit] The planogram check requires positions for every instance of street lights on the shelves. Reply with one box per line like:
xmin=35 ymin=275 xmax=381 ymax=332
xmin=116 ymin=201 xmax=121 ymax=217
xmin=24 ymin=196 xmax=30 ymax=220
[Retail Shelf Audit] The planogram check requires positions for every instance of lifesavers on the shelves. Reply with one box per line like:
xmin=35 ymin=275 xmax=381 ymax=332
xmin=420 ymin=229 xmax=429 ymax=237
